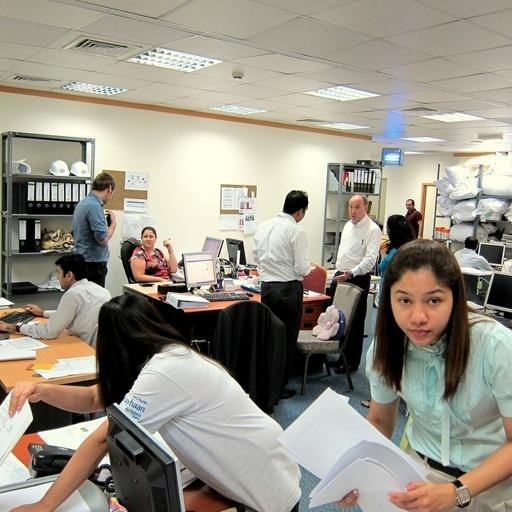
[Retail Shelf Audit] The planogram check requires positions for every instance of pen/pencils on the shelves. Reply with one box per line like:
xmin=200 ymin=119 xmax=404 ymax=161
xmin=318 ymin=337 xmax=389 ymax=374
xmin=217 ymin=272 xmax=223 ymax=279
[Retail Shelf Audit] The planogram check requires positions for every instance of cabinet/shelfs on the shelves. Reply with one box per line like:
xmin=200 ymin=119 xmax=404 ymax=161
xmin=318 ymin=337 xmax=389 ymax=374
xmin=319 ymin=161 xmax=382 ymax=275
xmin=0 ymin=132 xmax=96 ymax=302
xmin=431 ymin=190 xmax=512 ymax=261
xmin=299 ymin=294 xmax=331 ymax=331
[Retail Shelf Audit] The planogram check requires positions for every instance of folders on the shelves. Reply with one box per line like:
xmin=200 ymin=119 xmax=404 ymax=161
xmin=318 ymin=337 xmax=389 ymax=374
xmin=29 ymin=219 xmax=41 ymax=252
xmin=346 ymin=169 xmax=376 ymax=193
xmin=12 ymin=181 xmax=93 ymax=214
xmin=18 ymin=220 xmax=29 ymax=253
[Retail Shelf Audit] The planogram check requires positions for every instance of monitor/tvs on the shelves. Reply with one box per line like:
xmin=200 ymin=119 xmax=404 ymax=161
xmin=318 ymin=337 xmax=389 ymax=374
xmin=477 ymin=242 xmax=506 ymax=267
xmin=202 ymin=237 xmax=224 ymax=257
xmin=182 ymin=251 xmax=217 ymax=294
xmin=105 ymin=402 xmax=185 ymax=512
xmin=226 ymin=238 xmax=247 ymax=266
xmin=484 ymin=272 xmax=512 ymax=320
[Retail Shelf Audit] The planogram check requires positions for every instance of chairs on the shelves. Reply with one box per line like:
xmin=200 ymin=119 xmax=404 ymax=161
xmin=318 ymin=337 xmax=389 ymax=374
xmin=295 ymin=280 xmax=362 ymax=395
xmin=120 ymin=239 xmax=146 ymax=282
xmin=190 ymin=301 xmax=286 ymax=415
xmin=301 ymin=263 xmax=328 ymax=295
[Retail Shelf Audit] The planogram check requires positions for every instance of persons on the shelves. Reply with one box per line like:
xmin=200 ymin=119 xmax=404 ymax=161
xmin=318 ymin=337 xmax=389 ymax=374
xmin=361 ymin=215 xmax=414 ymax=407
xmin=453 ymin=235 xmax=493 ymax=271
xmin=72 ymin=173 xmax=117 ymax=288
xmin=258 ymin=189 xmax=312 ymax=400
xmin=329 ymin=196 xmax=381 ymax=374
xmin=5 ymin=290 xmax=301 ymax=511
xmin=404 ymin=198 xmax=424 ymax=239
xmin=333 ymin=238 xmax=511 ymax=511
xmin=130 ymin=226 xmax=177 ymax=283
xmin=1 ymin=254 xmax=113 ymax=350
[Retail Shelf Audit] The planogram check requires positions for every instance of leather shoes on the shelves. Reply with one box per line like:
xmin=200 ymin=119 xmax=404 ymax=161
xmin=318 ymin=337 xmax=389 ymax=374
xmin=280 ymin=390 xmax=296 ymax=399
xmin=335 ymin=365 xmax=350 ymax=374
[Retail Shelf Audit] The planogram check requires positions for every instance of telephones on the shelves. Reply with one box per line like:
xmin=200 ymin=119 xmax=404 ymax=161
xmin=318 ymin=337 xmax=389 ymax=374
xmin=27 ymin=443 xmax=100 ymax=481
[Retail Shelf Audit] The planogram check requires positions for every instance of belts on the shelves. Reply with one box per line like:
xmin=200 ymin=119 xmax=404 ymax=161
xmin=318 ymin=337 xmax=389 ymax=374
xmin=418 ymin=453 xmax=463 ymax=478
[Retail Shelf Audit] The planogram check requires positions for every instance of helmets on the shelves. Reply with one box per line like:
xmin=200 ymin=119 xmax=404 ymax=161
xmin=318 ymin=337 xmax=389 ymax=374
xmin=71 ymin=161 xmax=88 ymax=177
xmin=50 ymin=160 xmax=69 ymax=176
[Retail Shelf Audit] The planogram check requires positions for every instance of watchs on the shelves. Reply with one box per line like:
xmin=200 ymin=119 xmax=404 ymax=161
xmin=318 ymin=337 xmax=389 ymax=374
xmin=452 ymin=480 xmax=471 ymax=508
xmin=16 ymin=322 xmax=23 ymax=333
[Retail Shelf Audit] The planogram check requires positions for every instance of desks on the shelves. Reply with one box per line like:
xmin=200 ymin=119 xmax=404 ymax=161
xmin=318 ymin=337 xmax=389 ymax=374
xmin=0 ymin=431 xmax=237 ymax=511
xmin=121 ymin=276 xmax=330 ymax=376
xmin=0 ymin=307 xmax=100 ymax=435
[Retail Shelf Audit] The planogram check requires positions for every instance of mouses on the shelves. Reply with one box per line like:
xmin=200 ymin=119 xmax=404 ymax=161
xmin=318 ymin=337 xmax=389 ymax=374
xmin=245 ymin=292 xmax=253 ymax=297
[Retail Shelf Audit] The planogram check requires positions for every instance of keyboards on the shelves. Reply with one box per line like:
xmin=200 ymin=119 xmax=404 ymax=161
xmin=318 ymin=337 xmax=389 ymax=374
xmin=486 ymin=312 xmax=512 ymax=329
xmin=0 ymin=310 xmax=34 ymax=324
xmin=198 ymin=292 xmax=249 ymax=302
